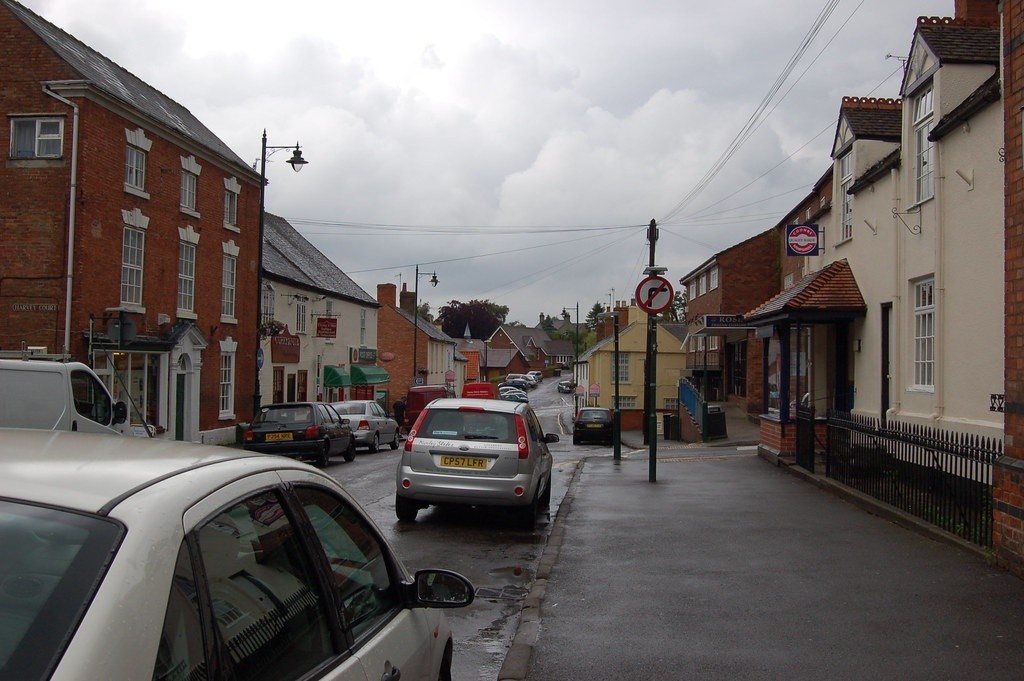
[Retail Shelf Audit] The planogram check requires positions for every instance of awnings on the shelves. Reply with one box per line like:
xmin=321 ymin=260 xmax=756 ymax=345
xmin=324 ymin=365 xmax=352 ymax=388
xmin=350 ymin=366 xmax=390 ymax=387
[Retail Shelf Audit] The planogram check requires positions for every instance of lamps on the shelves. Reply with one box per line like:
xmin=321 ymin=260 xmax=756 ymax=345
xmin=263 ymin=282 xmax=275 ymax=293
xmin=312 ymin=295 xmax=327 ymax=302
xmin=281 ymin=293 xmax=309 ymax=301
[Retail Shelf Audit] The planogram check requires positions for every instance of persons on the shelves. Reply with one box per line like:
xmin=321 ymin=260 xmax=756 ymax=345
xmin=393 ymin=396 xmax=407 ymax=438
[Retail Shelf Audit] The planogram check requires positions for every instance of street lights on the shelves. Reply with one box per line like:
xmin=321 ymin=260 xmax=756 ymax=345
xmin=251 ymin=128 xmax=309 ymax=414
xmin=560 ymin=301 xmax=579 ymax=419
xmin=635 ymin=266 xmax=674 ymax=482
xmin=595 ymin=311 xmax=621 ymax=460
xmin=413 ymin=264 xmax=440 ymax=376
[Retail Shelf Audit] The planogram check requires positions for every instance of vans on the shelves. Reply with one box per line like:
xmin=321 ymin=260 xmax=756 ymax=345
xmin=558 ymin=380 xmax=572 ymax=393
xmin=404 ymin=385 xmax=456 ymax=436
xmin=0 ymin=341 xmax=136 ymax=436
xmin=507 ymin=370 xmax=543 ymax=387
xmin=462 ymin=382 xmax=501 ymax=399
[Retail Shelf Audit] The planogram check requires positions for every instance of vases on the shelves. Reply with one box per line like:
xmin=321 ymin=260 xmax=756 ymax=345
xmin=265 ymin=327 xmax=285 ymax=335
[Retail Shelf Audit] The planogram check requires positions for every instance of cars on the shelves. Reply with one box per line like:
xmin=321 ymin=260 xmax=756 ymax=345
xmin=572 ymin=406 xmax=614 ymax=444
xmin=330 ymin=400 xmax=399 ymax=452
xmin=243 ymin=402 xmax=356 ymax=466
xmin=562 ymin=362 xmax=570 ymax=370
xmin=396 ymin=397 xmax=560 ymax=521
xmin=498 ymin=380 xmax=528 ymax=402
xmin=0 ymin=427 xmax=475 ymax=680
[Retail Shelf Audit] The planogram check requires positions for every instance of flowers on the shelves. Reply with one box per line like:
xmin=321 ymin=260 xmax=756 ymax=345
xmin=259 ymin=318 xmax=285 ymax=347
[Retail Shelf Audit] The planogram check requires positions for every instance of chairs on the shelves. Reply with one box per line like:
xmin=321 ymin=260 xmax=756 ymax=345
xmin=437 ymin=414 xmax=465 ymax=435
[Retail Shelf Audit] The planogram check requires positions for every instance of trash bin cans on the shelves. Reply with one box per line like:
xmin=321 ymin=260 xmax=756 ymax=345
xmin=663 ymin=413 xmax=672 ymax=440
xmin=708 ymin=405 xmax=728 ymax=439
xmin=670 ymin=415 xmax=679 ymax=440
